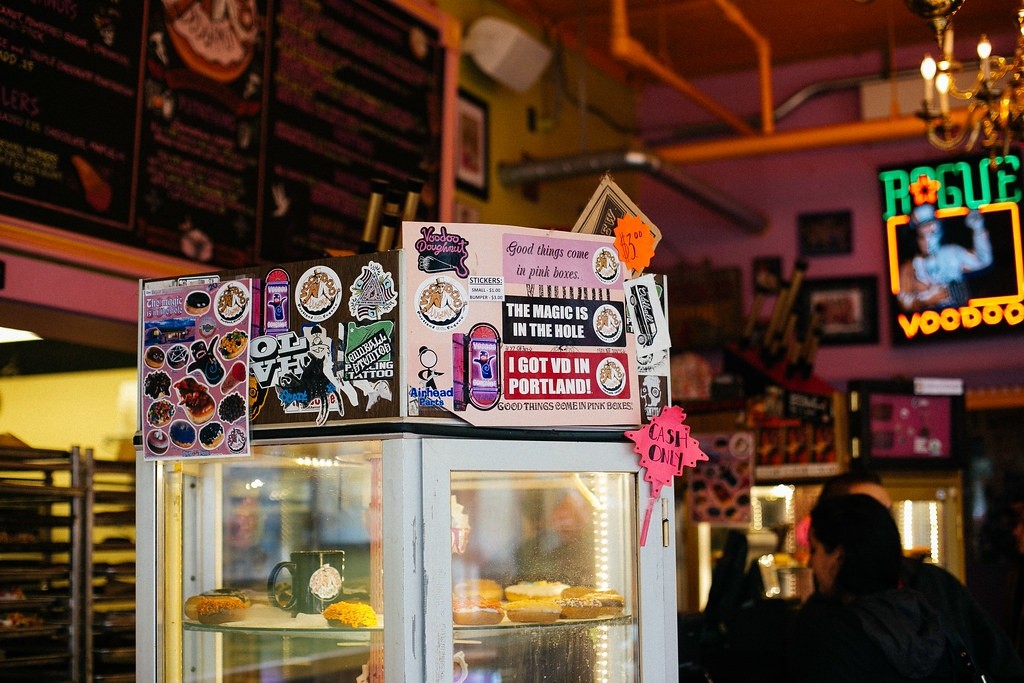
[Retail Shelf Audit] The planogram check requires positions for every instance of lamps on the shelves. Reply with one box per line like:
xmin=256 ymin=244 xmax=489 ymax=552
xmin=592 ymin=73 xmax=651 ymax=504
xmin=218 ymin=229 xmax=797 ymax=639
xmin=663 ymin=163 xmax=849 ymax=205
xmin=914 ymin=0 xmax=1024 ymax=152
xmin=461 ymin=16 xmax=552 ymax=94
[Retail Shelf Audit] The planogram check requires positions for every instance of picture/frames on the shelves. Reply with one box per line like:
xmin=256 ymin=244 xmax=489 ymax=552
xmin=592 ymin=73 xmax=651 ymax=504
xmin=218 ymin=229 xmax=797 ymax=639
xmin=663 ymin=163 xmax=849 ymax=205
xmin=751 ymin=255 xmax=781 ymax=293
xmin=791 ymin=270 xmax=881 ymax=345
xmin=795 ymin=208 xmax=854 ymax=257
xmin=454 ymin=85 xmax=492 ymax=201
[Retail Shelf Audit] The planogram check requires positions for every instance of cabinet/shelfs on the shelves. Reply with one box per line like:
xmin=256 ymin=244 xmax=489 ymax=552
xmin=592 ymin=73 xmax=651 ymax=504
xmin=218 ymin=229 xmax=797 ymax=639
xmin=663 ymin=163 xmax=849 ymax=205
xmin=0 ymin=444 xmax=136 ymax=683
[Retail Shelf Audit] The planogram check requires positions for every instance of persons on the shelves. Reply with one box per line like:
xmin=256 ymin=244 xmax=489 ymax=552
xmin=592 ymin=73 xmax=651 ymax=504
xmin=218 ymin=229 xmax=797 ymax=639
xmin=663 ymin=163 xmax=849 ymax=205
xmin=705 ymin=530 xmax=764 ymax=615
xmin=797 ymin=467 xmax=1024 ymax=683
xmin=899 ymin=203 xmax=992 ymax=311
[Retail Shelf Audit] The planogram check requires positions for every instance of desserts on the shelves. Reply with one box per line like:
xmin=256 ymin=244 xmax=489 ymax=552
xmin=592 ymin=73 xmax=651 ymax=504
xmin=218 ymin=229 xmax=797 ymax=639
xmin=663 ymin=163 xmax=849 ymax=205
xmin=184 ymin=588 xmax=251 ymax=625
xmin=321 ymin=602 xmax=379 ymax=629
xmin=451 ymin=577 xmax=625 ymax=625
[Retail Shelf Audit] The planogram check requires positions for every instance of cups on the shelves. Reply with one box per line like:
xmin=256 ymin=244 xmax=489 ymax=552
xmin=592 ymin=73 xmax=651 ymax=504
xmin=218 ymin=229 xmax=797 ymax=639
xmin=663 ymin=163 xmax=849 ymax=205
xmin=268 ymin=550 xmax=343 ymax=618
